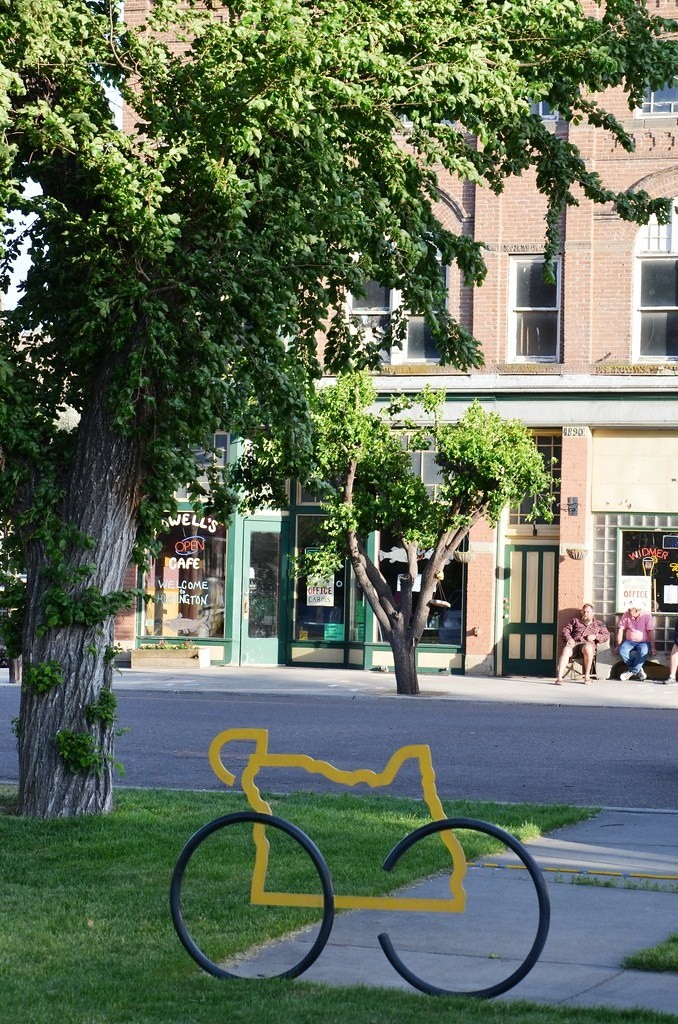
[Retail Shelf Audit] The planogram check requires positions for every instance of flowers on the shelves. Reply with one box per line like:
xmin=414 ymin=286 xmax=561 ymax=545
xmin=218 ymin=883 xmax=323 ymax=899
xmin=136 ymin=638 xmax=200 ymax=651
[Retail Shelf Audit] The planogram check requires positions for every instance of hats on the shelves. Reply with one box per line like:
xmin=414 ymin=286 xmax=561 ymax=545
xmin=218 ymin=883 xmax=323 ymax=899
xmin=627 ymin=599 xmax=643 ymax=609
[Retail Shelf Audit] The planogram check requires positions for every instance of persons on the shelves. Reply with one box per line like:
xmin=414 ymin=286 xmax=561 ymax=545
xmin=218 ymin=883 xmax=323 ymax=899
xmin=555 ymin=603 xmax=610 ymax=685
xmin=613 ymin=600 xmax=657 ymax=682
xmin=663 ymin=619 xmax=678 ymax=684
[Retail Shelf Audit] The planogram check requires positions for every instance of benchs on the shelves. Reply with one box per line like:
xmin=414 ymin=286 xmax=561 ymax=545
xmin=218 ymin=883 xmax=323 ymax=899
xmin=595 ymin=633 xmax=678 ymax=681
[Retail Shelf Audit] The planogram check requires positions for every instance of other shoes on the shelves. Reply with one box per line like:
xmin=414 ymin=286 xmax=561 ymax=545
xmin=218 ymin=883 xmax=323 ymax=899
xmin=584 ymin=679 xmax=591 ymax=685
xmin=556 ymin=680 xmax=563 ymax=686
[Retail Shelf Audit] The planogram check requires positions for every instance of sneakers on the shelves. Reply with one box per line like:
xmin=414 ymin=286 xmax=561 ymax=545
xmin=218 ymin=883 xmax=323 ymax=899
xmin=638 ymin=667 xmax=646 ymax=681
xmin=620 ymin=671 xmax=633 ymax=681
xmin=663 ymin=677 xmax=676 ymax=684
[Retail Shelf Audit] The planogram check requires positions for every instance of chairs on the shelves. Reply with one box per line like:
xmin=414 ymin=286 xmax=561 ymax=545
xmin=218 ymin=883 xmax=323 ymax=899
xmin=561 ymin=635 xmax=601 ymax=683
